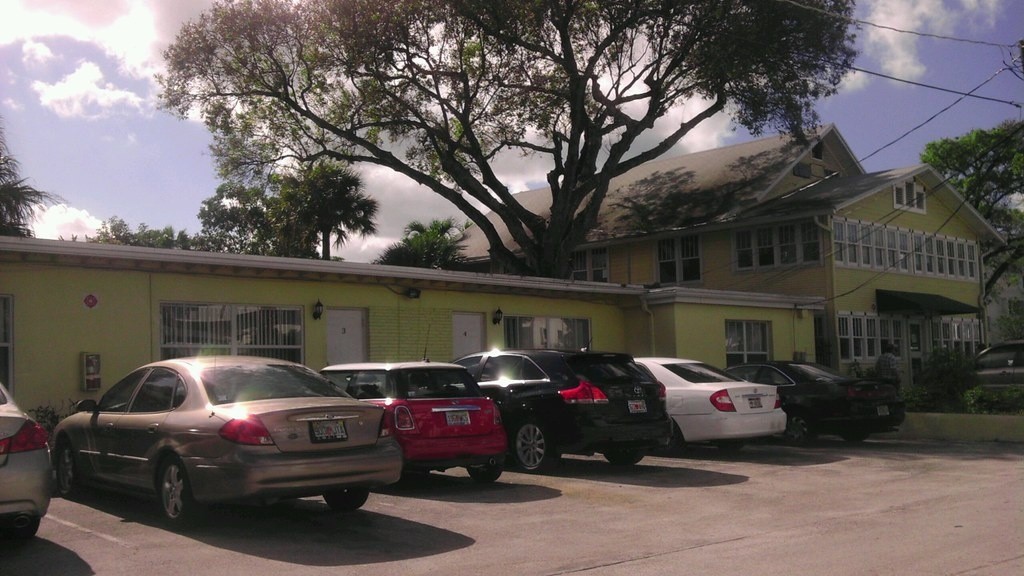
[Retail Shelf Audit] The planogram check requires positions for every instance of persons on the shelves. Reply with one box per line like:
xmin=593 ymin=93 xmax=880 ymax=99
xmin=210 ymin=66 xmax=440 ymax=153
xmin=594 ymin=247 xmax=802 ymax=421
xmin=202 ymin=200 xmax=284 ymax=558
xmin=876 ymin=344 xmax=902 ymax=387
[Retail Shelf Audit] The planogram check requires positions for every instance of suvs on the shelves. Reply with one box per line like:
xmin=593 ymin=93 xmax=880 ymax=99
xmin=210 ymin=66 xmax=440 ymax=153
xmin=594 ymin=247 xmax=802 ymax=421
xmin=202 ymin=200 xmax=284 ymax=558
xmin=450 ymin=348 xmax=673 ymax=476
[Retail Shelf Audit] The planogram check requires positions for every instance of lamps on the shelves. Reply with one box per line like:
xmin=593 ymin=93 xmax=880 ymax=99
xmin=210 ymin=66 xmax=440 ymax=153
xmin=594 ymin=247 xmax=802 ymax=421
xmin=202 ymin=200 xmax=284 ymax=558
xmin=313 ymin=298 xmax=324 ymax=320
xmin=492 ymin=307 xmax=503 ymax=325
xmin=405 ymin=287 xmax=421 ymax=298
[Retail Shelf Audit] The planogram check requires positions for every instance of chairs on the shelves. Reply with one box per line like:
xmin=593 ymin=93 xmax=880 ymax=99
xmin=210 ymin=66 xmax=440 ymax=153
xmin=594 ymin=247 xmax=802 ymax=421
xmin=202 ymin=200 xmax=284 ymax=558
xmin=357 ymin=375 xmax=386 ymax=399
xmin=752 ymin=369 xmax=775 ymax=385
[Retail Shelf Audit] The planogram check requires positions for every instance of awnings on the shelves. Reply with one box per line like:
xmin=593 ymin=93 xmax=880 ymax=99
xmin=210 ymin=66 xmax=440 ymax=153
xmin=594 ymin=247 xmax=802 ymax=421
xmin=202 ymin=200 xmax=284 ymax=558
xmin=876 ymin=289 xmax=981 ymax=318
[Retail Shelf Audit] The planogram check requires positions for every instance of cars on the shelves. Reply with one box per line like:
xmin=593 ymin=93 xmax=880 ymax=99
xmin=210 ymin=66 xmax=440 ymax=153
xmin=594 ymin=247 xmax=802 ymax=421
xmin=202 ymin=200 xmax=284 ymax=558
xmin=50 ymin=355 xmax=406 ymax=531
xmin=936 ymin=340 xmax=1024 ymax=404
xmin=321 ymin=363 xmax=509 ymax=489
xmin=0 ymin=383 xmax=52 ymax=549
xmin=631 ymin=356 xmax=788 ymax=458
xmin=724 ymin=359 xmax=906 ymax=447
